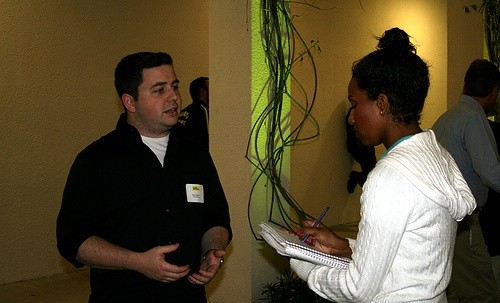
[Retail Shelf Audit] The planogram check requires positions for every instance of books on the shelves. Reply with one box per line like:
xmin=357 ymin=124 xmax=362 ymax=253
xmin=257 ymin=222 xmax=352 ymax=270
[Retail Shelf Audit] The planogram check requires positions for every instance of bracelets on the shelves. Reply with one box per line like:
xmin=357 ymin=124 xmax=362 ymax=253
xmin=201 ymin=249 xmax=224 ymax=269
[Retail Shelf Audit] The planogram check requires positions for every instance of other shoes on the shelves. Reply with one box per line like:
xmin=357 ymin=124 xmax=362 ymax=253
xmin=347 ymin=171 xmax=358 ymax=194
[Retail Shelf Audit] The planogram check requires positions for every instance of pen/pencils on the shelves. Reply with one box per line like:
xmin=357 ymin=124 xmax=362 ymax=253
xmin=302 ymin=206 xmax=330 ymax=243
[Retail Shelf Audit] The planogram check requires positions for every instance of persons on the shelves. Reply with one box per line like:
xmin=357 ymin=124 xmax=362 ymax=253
xmin=171 ymin=77 xmax=209 ymax=154
xmin=346 ymin=105 xmax=377 ymax=194
xmin=432 ymin=58 xmax=500 ymax=303
xmin=281 ymin=26 xmax=477 ymax=303
xmin=56 ymin=51 xmax=234 ymax=303
